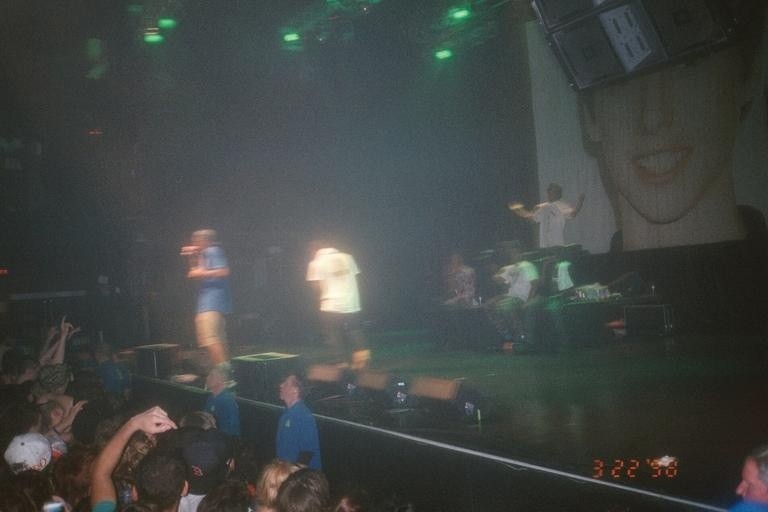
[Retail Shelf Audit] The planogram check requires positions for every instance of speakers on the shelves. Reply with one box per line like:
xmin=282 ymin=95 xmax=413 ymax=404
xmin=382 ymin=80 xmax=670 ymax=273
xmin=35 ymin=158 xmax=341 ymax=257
xmin=133 ymin=343 xmax=182 ymax=379
xmin=230 ymin=352 xmax=301 ymax=401
xmin=624 ymin=305 xmax=672 ymax=341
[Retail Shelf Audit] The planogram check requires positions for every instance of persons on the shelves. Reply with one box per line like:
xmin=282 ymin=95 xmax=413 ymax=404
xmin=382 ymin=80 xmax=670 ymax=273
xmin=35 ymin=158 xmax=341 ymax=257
xmin=482 ymin=248 xmax=541 ymax=348
xmin=507 ymin=179 xmax=586 ymax=257
xmin=305 ymin=238 xmax=370 ymax=371
xmin=436 ymin=253 xmax=479 ymax=310
xmin=1 ymin=314 xmax=370 ymax=511
xmin=731 ymin=445 xmax=768 ymax=511
xmin=572 ymin=41 xmax=767 ymax=255
xmin=179 ymin=229 xmax=239 ymax=390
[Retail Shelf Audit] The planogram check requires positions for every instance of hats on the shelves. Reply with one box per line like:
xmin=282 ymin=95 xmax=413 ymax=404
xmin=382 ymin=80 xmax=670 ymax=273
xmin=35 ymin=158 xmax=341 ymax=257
xmin=182 ymin=429 xmax=232 ymax=495
xmin=3 ymin=430 xmax=54 ymax=473
